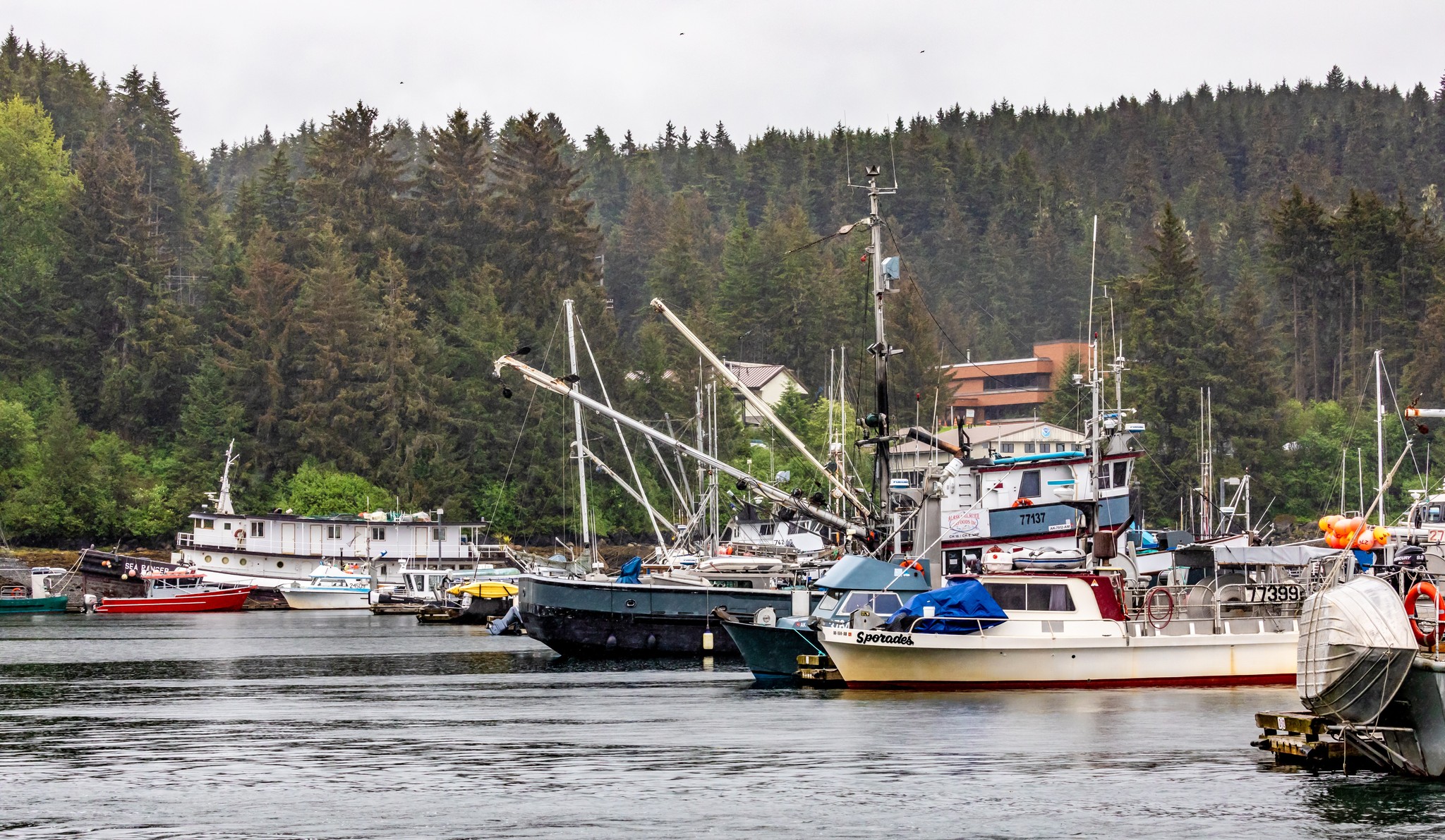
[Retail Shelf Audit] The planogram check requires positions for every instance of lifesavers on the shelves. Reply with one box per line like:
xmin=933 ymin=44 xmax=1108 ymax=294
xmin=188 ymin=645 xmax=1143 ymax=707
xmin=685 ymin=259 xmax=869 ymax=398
xmin=1012 ymin=497 xmax=1035 ymax=507
xmin=1404 ymin=582 xmax=1445 ymax=647
xmin=11 ymin=588 xmax=23 ymax=599
xmin=234 ymin=529 xmax=247 ymax=540
xmin=899 ymin=559 xmax=925 ymax=576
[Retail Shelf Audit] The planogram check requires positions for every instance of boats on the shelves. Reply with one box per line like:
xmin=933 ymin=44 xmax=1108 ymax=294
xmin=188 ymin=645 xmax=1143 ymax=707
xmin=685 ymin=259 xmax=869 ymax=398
xmin=0 ymin=566 xmax=258 ymax=613
xmin=277 ymin=111 xmax=1445 ymax=781
xmin=79 ymin=438 xmax=529 ymax=609
